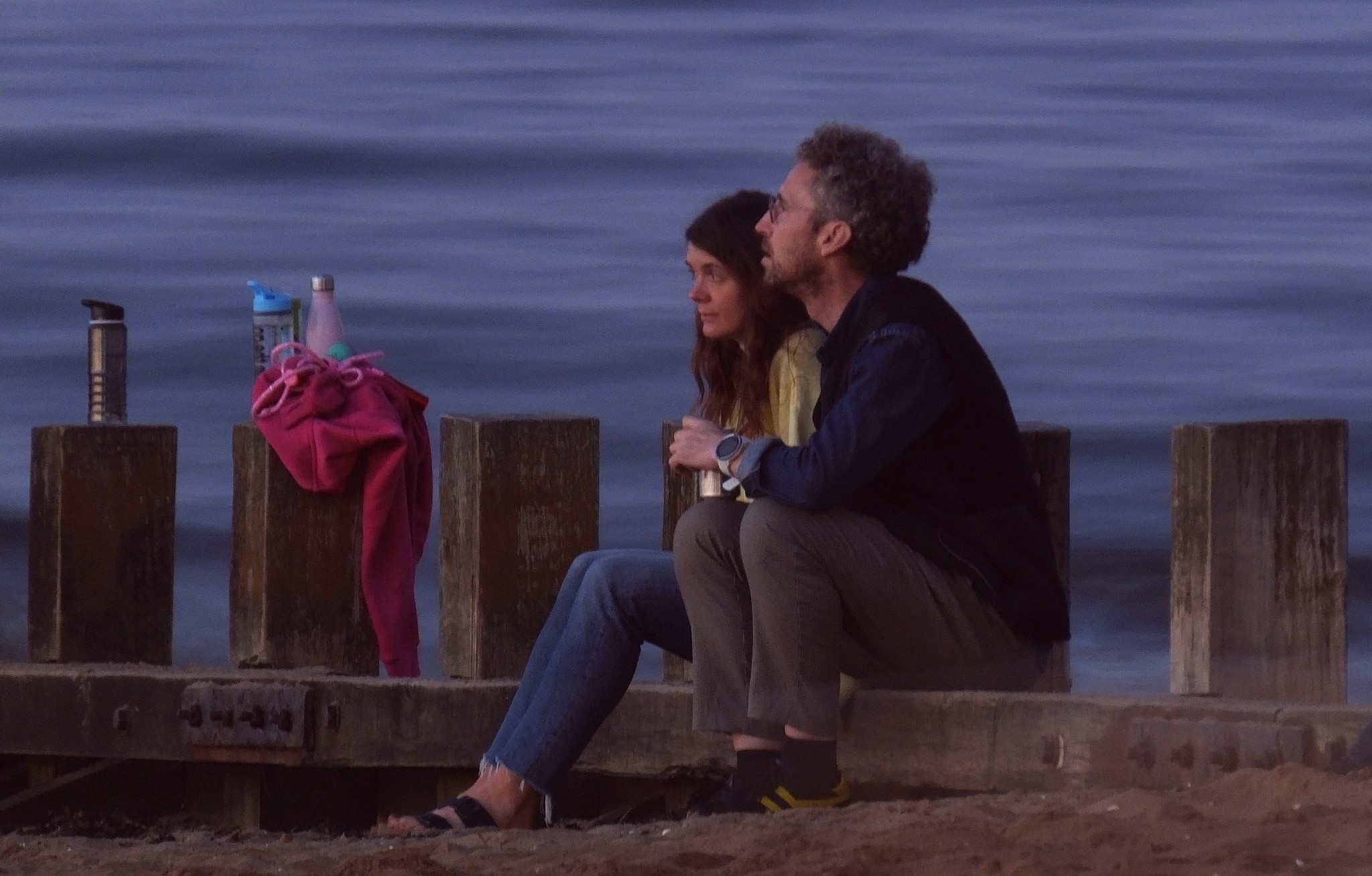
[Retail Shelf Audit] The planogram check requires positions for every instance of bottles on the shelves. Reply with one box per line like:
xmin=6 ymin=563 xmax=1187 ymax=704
xmin=81 ymin=299 xmax=129 ymax=425
xmin=305 ymin=273 xmax=344 ymax=361
xmin=247 ymin=280 xmax=300 ymax=377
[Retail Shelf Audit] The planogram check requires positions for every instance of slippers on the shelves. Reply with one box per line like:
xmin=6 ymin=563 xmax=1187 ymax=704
xmin=389 ymin=794 xmax=500 ymax=837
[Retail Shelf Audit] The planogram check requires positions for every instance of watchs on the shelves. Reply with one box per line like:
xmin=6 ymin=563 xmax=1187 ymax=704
xmin=716 ymin=432 xmax=754 ymax=491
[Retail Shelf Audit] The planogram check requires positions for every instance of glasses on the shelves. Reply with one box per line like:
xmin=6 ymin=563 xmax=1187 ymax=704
xmin=769 ymin=197 xmax=820 ymax=223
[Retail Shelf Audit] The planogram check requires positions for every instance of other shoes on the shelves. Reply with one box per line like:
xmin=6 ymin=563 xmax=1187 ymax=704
xmin=704 ymin=764 xmax=853 ymax=814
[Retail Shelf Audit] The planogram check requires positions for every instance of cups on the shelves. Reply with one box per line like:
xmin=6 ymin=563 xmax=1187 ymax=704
xmin=698 ymin=428 xmax=741 ymax=498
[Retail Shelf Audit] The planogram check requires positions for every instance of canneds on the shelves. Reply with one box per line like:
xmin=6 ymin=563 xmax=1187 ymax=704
xmin=699 ymin=428 xmax=741 ymax=499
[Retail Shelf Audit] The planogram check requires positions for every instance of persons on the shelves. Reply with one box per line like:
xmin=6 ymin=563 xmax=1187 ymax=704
xmin=666 ymin=120 xmax=1073 ymax=826
xmin=363 ymin=187 xmax=833 ymax=843
